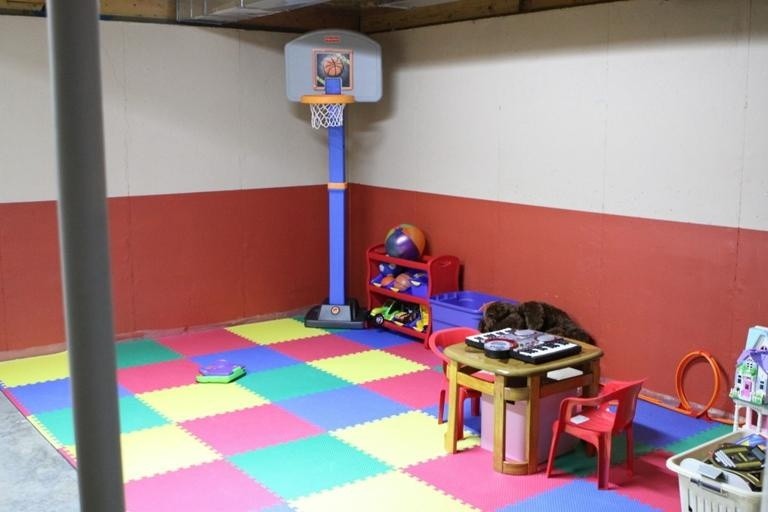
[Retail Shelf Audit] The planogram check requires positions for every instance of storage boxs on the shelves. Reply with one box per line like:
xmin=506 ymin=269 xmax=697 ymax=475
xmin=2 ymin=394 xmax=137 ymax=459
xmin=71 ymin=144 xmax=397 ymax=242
xmin=662 ymin=426 xmax=763 ymax=512
xmin=431 ymin=288 xmax=522 ymax=351
xmin=479 ymin=389 xmax=579 ymax=466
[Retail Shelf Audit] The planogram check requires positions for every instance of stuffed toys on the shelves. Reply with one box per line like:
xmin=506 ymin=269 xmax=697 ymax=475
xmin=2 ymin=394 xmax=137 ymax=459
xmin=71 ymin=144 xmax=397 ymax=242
xmin=479 ymin=300 xmax=595 ymax=346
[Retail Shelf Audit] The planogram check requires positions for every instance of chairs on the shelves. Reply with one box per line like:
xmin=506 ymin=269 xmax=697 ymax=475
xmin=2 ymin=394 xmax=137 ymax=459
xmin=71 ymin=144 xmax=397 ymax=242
xmin=428 ymin=324 xmax=486 ymax=441
xmin=545 ymin=379 xmax=647 ymax=490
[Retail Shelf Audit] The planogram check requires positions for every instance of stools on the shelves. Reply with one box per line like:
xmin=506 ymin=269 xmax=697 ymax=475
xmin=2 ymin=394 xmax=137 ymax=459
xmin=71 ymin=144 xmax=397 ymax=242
xmin=733 ymin=400 xmax=767 ymax=433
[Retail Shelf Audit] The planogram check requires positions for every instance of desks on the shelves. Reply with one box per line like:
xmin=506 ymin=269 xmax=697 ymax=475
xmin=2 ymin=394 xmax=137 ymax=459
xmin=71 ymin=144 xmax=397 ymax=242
xmin=444 ymin=328 xmax=604 ymax=476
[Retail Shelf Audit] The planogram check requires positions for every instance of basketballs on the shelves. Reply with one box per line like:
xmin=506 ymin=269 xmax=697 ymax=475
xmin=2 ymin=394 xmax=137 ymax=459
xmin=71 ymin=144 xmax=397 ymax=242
xmin=323 ymin=57 xmax=343 ymax=77
